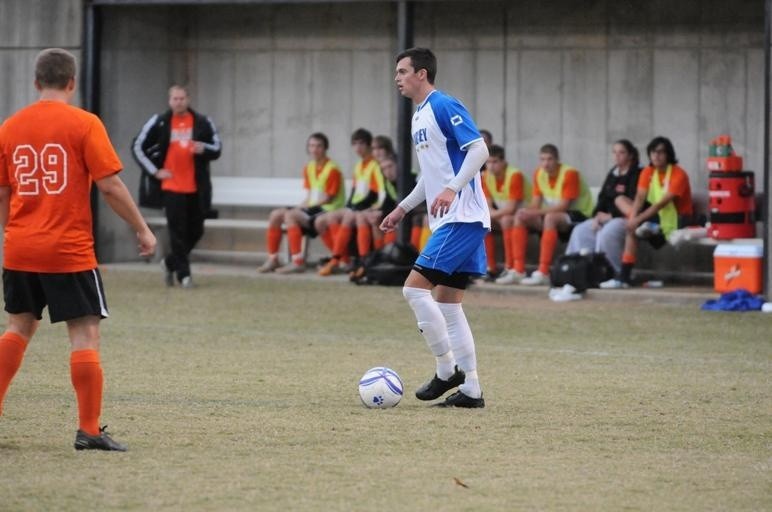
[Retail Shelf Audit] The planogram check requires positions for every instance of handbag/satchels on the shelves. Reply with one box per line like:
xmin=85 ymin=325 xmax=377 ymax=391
xmin=549 ymin=251 xmax=614 ymax=291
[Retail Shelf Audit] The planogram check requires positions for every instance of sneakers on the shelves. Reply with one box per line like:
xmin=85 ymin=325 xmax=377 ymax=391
xmin=339 ymin=259 xmax=354 ymax=270
xmin=320 ymin=261 xmax=338 ymax=275
xmin=520 ymin=270 xmax=551 ymax=285
xmin=635 ymin=221 xmax=661 ymax=237
xmin=275 ymin=262 xmax=305 ymax=273
xmin=415 ymin=364 xmax=465 ymax=401
xmin=599 ymin=279 xmax=628 ymax=289
xmin=495 ymin=268 xmax=527 ymax=284
xmin=473 ymin=272 xmax=496 ymax=284
xmin=256 ymin=259 xmax=279 ymax=272
xmin=74 ymin=424 xmax=126 ymax=452
xmin=181 ymin=276 xmax=198 ymax=288
xmin=432 ymin=390 xmax=485 ymax=409
xmin=350 ymin=266 xmax=365 ymax=280
xmin=552 ymin=283 xmax=582 ymax=302
xmin=160 ymin=259 xmax=174 ymax=287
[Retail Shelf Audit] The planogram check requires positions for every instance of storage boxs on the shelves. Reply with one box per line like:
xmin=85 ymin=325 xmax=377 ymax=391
xmin=713 ymin=238 xmax=765 ymax=292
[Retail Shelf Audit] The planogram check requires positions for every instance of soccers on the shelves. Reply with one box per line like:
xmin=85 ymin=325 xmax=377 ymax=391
xmin=358 ymin=367 xmax=404 ymax=409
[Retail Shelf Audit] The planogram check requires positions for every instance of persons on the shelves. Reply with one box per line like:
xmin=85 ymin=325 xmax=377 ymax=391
xmin=469 ymin=128 xmax=492 ymax=170
xmin=0 ymin=47 xmax=157 ymax=451
xmin=378 ymin=48 xmax=492 ymax=409
xmin=129 ymin=85 xmax=223 ymax=286
xmin=317 ymin=135 xmax=393 ymax=277
xmin=494 ymin=143 xmax=595 ymax=286
xmin=313 ymin=128 xmax=377 ymax=268
xmin=366 ymin=155 xmax=418 ymax=252
xmin=481 ymin=144 xmax=533 ymax=283
xmin=257 ymin=132 xmax=346 ymax=275
xmin=418 ymin=213 xmax=433 ymax=256
xmin=598 ymin=136 xmax=694 ymax=290
xmin=563 ymin=138 xmax=644 ymax=280
xmin=409 ymin=212 xmax=422 ymax=249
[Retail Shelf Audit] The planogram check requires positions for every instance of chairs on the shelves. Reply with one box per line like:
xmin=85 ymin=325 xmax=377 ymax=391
xmin=140 ymin=174 xmax=354 ymax=265
